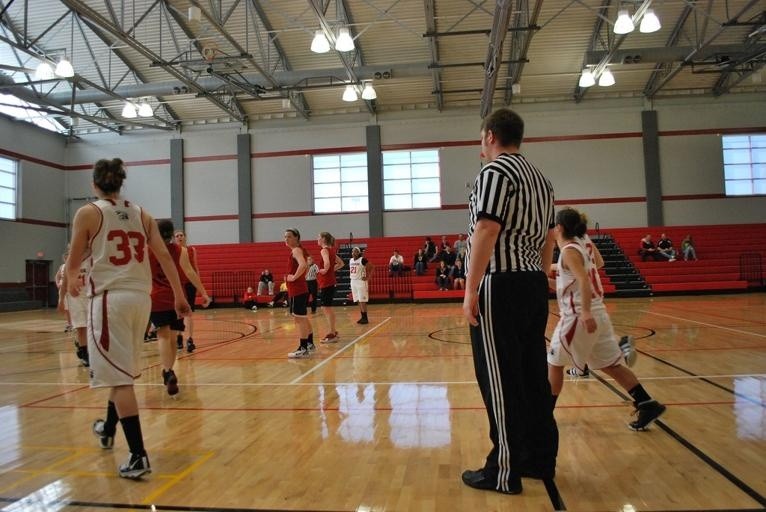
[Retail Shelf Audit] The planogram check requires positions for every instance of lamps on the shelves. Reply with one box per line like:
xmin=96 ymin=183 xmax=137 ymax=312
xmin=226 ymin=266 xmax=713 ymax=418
xmin=120 ymin=97 xmax=154 ymax=119
xmin=578 ymin=63 xmax=616 ymax=87
xmin=35 ymin=48 xmax=75 ymax=81
xmin=341 ymin=79 xmax=378 ymax=101
xmin=613 ymin=0 xmax=662 ymax=36
xmin=311 ymin=18 xmax=356 ymax=54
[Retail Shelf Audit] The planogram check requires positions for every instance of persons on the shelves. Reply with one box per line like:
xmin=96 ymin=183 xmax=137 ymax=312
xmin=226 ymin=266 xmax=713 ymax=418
xmin=545 ymin=207 xmax=667 ymax=432
xmin=50 ymin=219 xmax=213 ymax=397
xmin=456 ymin=107 xmax=559 ymax=495
xmin=545 ymin=213 xmax=638 ymax=379
xmin=638 ymin=233 xmax=661 ymax=262
xmin=282 ymin=226 xmax=317 ymax=359
xmin=237 ymin=254 xmax=320 ymax=318
xmin=315 ymin=231 xmax=346 ymax=344
xmin=655 ymin=233 xmax=677 ymax=262
xmin=386 ymin=231 xmax=469 ymax=291
xmin=346 ymin=245 xmax=376 ymax=326
xmin=679 ymin=232 xmax=697 ymax=262
xmin=64 ymin=156 xmax=196 ymax=480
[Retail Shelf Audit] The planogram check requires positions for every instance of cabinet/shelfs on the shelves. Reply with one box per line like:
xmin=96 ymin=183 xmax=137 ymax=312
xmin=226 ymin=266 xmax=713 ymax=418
xmin=25 ymin=259 xmax=53 ymax=306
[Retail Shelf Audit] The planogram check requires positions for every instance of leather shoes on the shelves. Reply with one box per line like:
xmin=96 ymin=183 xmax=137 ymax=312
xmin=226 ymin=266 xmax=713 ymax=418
xmin=462 ymin=468 xmax=496 ymax=491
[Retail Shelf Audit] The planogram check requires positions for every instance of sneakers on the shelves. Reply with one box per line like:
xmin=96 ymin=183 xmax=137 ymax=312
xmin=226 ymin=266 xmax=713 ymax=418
xmin=360 ymin=317 xmax=368 ymax=323
xmin=77 ymin=351 xmax=90 ymax=366
xmin=320 ymin=333 xmax=338 ymax=344
xmin=93 ymin=418 xmax=113 ymax=449
xmin=118 ymin=450 xmax=151 ymax=478
xmin=176 ymin=334 xmax=183 ymax=349
xmin=149 ymin=331 xmax=157 ymax=339
xmin=567 ymin=367 xmax=589 ymax=378
xmin=186 ymin=338 xmax=195 ymax=352
xmin=306 ymin=342 xmax=316 ymax=352
xmin=287 ymin=345 xmax=310 ymax=359
xmin=619 ymin=335 xmax=637 ymax=368
xmin=628 ymin=398 xmax=666 ymax=431
xmin=161 ymin=370 xmax=178 ymax=395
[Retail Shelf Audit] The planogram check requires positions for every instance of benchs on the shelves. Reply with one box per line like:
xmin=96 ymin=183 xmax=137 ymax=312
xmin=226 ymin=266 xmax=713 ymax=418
xmin=0 ymin=286 xmax=43 ymax=312
